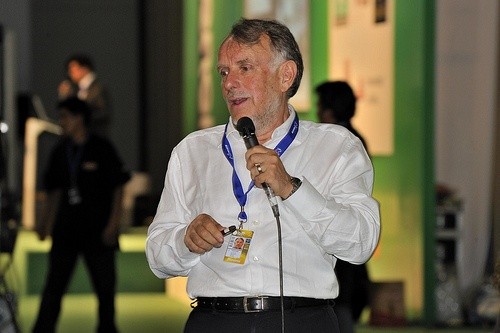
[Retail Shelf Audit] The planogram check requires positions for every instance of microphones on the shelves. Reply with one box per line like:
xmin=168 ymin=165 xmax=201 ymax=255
xmin=237 ymin=117 xmax=279 ymax=218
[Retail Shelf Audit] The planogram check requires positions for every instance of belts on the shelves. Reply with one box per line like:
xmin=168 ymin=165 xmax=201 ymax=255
xmin=198 ymin=296 xmax=327 ymax=313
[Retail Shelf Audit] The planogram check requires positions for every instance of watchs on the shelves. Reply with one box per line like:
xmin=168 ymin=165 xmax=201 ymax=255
xmin=281 ymin=176 xmax=301 ymax=202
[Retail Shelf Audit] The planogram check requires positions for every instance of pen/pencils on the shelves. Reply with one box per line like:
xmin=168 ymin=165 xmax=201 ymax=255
xmin=189 ymin=225 xmax=237 ymax=253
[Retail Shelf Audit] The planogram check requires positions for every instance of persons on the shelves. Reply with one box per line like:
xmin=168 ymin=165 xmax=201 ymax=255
xmin=0 ymin=55 xmax=373 ymax=333
xmin=143 ymin=15 xmax=381 ymax=333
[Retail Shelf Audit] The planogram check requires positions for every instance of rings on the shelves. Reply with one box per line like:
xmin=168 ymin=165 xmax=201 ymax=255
xmin=257 ymin=166 xmax=262 ymax=174
